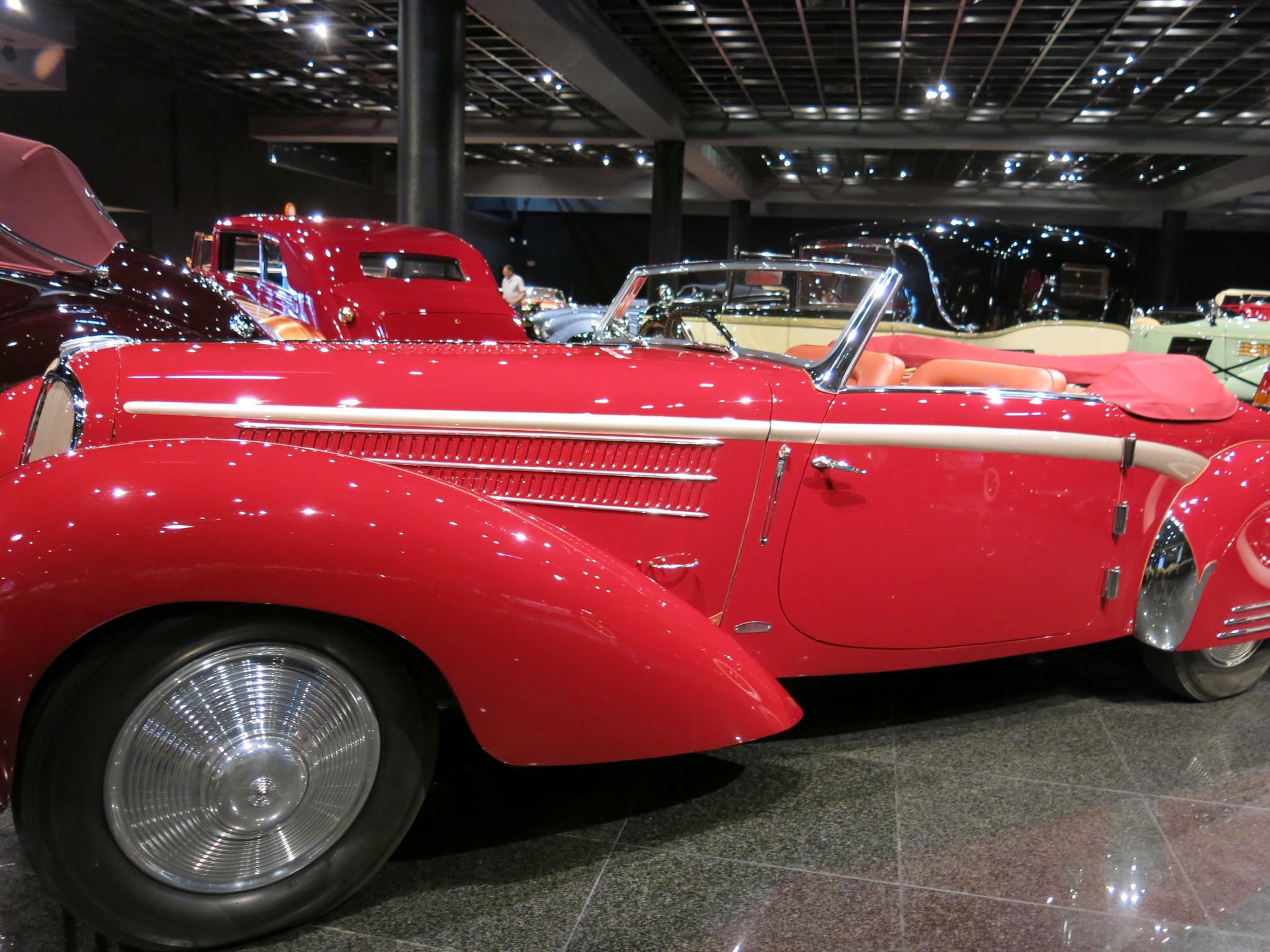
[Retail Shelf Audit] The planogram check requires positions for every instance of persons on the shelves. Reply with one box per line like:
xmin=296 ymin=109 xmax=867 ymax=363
xmin=1021 ymin=265 xmax=1043 ymax=307
xmin=501 ymin=264 xmax=526 ymax=314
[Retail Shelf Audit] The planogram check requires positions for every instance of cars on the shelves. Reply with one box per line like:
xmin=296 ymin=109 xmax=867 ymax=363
xmin=0 ymin=259 xmax=1268 ymax=951
xmin=196 ymin=217 xmax=531 ymax=344
xmin=0 ymin=134 xmax=275 ymax=393
xmin=532 ymin=286 xmax=790 ymax=346
xmin=1131 ymin=287 xmax=1270 ymax=402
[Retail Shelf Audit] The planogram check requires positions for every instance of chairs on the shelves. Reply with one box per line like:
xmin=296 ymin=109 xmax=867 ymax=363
xmin=784 ymin=341 xmax=906 ymax=387
xmin=908 ymin=357 xmax=1067 ymax=393
